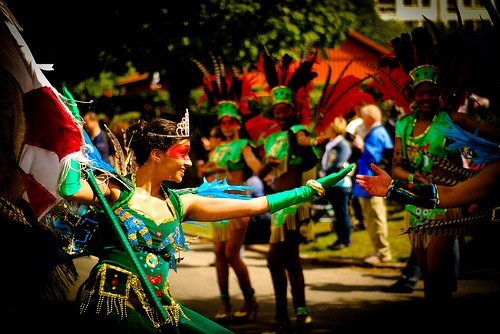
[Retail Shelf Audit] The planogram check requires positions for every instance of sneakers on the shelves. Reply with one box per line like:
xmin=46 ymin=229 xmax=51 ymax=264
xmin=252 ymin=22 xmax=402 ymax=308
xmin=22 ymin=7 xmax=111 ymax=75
xmin=364 ymin=251 xmax=393 ymax=264
xmin=328 ymin=240 xmax=352 ymax=250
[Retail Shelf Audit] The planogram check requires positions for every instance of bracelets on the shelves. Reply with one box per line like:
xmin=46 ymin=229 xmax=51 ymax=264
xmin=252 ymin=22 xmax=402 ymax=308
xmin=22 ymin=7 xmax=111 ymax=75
xmin=306 ymin=180 xmax=325 ymax=197
xmin=310 ymin=138 xmax=315 ymax=146
xmin=408 ymin=174 xmax=414 ymax=184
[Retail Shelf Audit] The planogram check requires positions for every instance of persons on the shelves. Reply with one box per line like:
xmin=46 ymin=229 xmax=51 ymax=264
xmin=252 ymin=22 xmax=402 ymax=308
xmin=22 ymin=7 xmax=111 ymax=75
xmin=356 ymin=83 xmax=500 ymax=334
xmin=54 ymin=106 xmax=354 ymax=334
xmin=195 ymin=102 xmax=395 ymax=326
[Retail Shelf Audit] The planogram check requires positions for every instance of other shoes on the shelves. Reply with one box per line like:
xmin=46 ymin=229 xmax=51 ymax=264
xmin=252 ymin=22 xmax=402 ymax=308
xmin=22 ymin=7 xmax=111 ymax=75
xmin=381 ymin=279 xmax=414 ymax=294
xmin=214 ymin=295 xmax=233 ymax=320
xmin=232 ymin=300 xmax=259 ymax=322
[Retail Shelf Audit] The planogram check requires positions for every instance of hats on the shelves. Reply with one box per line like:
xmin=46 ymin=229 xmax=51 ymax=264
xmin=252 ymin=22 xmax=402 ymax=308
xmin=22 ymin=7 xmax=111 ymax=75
xmin=262 ymin=53 xmax=318 ymax=106
xmin=203 ymin=75 xmax=243 ymax=123
xmin=391 ymin=23 xmax=445 ymax=87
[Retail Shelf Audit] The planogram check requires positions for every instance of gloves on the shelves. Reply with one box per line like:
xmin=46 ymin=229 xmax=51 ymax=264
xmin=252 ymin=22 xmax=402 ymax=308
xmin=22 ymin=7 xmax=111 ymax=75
xmin=266 ymin=163 xmax=354 ymax=214
xmin=58 ymin=85 xmax=82 ymax=197
